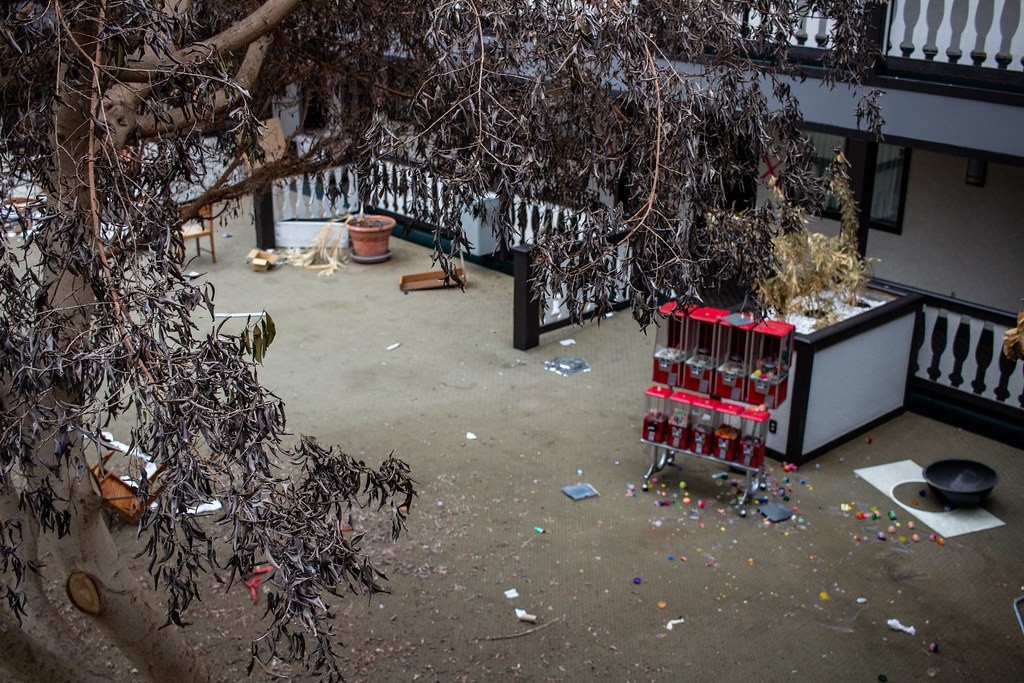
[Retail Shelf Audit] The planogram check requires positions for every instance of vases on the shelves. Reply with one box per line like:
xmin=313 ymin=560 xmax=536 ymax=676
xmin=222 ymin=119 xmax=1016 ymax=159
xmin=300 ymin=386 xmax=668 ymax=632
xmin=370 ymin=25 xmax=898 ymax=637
xmin=345 ymin=215 xmax=397 ymax=257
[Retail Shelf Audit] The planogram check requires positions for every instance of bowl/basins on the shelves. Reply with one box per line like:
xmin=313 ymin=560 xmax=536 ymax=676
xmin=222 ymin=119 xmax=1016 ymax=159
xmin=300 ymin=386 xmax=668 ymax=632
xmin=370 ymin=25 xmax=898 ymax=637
xmin=922 ymin=458 xmax=999 ymax=506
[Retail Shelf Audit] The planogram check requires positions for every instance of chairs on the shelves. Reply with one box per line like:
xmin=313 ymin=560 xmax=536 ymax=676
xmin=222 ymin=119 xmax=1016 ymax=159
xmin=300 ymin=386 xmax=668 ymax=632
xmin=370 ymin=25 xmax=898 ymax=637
xmin=169 ymin=203 xmax=217 ymax=263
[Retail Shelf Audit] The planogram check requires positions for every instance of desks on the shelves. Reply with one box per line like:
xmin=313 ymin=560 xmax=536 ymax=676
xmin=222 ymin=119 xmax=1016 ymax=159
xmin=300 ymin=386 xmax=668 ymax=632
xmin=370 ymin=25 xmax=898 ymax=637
xmin=90 ymin=450 xmax=168 ymax=526
xmin=722 ymin=283 xmax=923 ymax=468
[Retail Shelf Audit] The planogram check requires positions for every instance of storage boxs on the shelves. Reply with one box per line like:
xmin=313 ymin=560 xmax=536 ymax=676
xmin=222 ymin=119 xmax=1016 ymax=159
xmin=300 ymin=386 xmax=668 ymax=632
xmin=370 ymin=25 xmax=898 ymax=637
xmin=248 ymin=248 xmax=278 ymax=272
